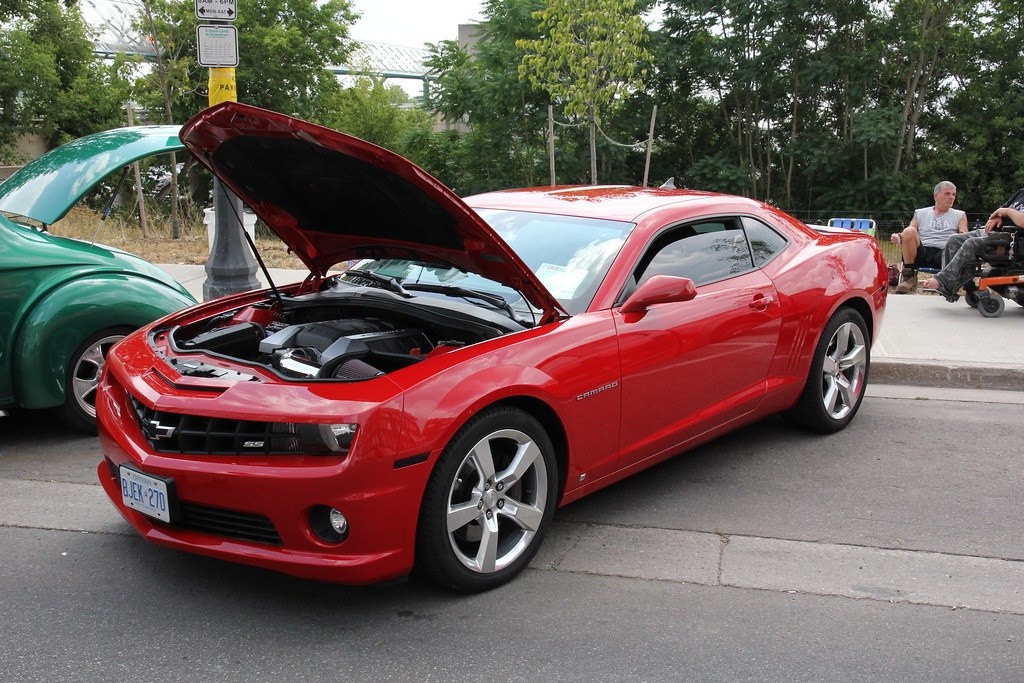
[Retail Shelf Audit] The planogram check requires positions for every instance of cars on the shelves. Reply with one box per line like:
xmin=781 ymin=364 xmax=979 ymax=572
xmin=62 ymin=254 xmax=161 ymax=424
xmin=0 ymin=125 xmax=202 ymax=437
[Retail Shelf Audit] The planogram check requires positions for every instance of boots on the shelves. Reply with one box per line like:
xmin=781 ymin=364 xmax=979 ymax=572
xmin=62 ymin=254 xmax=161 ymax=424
xmin=895 ymin=268 xmax=919 ymax=294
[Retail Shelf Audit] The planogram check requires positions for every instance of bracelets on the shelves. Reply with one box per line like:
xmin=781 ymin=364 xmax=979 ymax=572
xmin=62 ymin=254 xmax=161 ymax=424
xmin=898 ymin=233 xmax=902 ymax=244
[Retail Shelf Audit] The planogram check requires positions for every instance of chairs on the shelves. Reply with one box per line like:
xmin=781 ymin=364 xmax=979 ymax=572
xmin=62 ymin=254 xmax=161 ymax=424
xmin=897 ymin=232 xmax=950 ymax=290
xmin=827 ymin=218 xmax=876 ymax=238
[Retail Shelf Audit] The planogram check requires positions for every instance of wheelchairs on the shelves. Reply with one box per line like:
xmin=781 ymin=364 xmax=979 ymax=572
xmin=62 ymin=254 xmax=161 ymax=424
xmin=922 ymin=219 xmax=1024 ymax=318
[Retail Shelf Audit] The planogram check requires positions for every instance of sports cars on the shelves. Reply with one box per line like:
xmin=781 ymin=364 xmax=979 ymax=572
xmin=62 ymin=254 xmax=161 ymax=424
xmin=94 ymin=101 xmax=891 ymax=598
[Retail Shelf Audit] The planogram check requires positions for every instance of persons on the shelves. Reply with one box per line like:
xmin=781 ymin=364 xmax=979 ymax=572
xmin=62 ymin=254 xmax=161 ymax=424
xmin=921 ymin=187 xmax=1024 ymax=297
xmin=891 ymin=181 xmax=969 ymax=294
